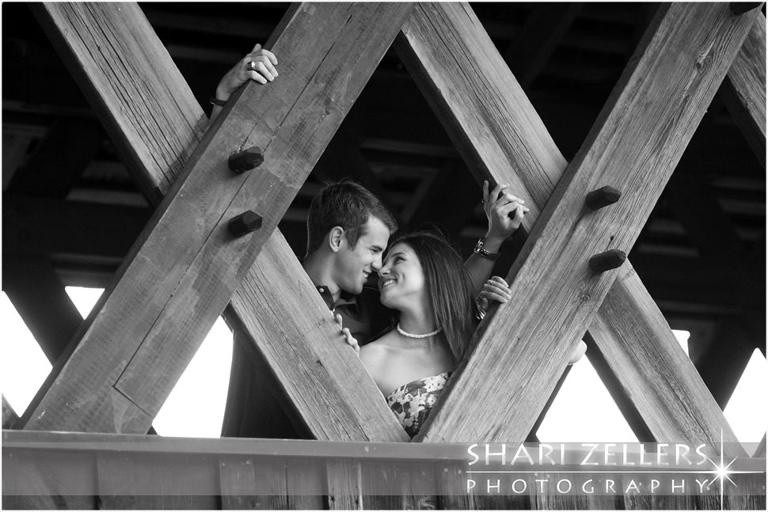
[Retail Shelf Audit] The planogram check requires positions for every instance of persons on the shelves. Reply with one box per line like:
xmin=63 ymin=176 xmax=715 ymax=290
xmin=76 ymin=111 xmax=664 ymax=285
xmin=207 ymin=39 xmax=532 ymax=446
xmin=329 ymin=230 xmax=588 ymax=440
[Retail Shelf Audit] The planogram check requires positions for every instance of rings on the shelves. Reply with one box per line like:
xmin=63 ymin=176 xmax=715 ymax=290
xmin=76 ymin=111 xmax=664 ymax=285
xmin=489 ymin=280 xmax=496 ymax=285
xmin=246 ymin=60 xmax=257 ymax=72
xmin=480 ymin=199 xmax=489 ymax=204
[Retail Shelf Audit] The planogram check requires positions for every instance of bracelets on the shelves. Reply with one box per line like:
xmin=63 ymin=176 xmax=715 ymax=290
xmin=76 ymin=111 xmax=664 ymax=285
xmin=209 ymin=97 xmax=228 ymax=107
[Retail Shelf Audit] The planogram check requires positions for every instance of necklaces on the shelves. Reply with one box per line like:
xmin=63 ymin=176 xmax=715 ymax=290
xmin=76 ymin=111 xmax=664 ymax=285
xmin=394 ymin=322 xmax=443 ymax=339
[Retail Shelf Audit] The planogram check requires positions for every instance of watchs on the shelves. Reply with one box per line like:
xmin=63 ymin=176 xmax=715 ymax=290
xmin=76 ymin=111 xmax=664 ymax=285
xmin=472 ymin=236 xmax=501 ymax=262
